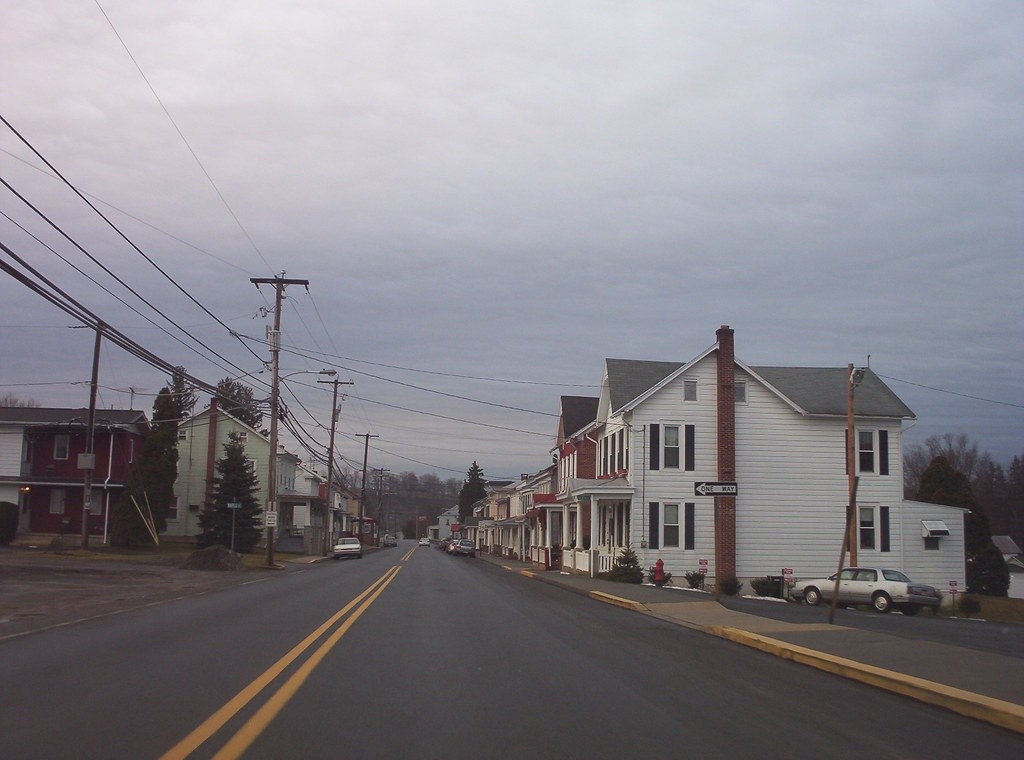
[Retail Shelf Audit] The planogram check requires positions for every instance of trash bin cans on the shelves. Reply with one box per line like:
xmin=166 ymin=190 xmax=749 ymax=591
xmin=767 ymin=575 xmax=783 ymax=598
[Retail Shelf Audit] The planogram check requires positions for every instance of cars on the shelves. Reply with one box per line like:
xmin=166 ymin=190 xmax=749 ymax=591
xmin=385 ymin=536 xmax=398 ymax=547
xmin=441 ymin=538 xmax=475 ymax=556
xmin=419 ymin=537 xmax=430 ymax=547
xmin=333 ymin=537 xmax=362 ymax=559
xmin=792 ymin=565 xmax=939 ymax=616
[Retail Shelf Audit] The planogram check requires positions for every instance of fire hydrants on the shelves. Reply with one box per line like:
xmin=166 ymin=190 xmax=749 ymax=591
xmin=655 ymin=558 xmax=665 ymax=588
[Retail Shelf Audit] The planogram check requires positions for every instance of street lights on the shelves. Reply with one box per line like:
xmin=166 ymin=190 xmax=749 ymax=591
xmin=375 ymin=491 xmax=398 ymax=546
xmin=266 ymin=369 xmax=337 ymax=564
xmin=848 ymin=367 xmax=866 ymax=567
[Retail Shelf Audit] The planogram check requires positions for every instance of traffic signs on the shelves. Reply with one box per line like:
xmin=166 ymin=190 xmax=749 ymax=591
xmin=694 ymin=482 xmax=738 ymax=496
